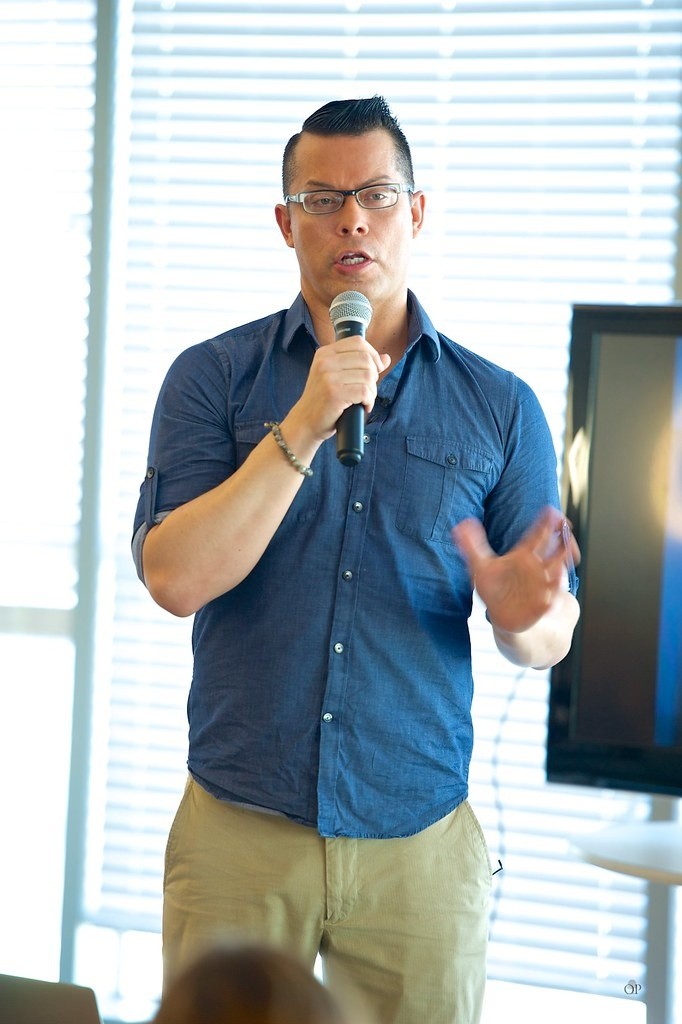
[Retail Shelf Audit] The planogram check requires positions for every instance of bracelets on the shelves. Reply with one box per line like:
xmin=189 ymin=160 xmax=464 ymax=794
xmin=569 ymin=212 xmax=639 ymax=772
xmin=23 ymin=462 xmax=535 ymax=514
xmin=265 ymin=422 xmax=314 ymax=477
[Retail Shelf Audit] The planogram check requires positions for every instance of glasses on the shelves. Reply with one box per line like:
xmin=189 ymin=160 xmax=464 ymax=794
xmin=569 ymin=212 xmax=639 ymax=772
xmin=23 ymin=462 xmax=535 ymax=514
xmin=285 ymin=183 xmax=416 ymax=216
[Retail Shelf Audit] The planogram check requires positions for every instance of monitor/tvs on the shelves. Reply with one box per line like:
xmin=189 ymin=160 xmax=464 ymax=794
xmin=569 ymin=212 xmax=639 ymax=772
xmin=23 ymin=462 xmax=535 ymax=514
xmin=546 ymin=303 xmax=682 ymax=798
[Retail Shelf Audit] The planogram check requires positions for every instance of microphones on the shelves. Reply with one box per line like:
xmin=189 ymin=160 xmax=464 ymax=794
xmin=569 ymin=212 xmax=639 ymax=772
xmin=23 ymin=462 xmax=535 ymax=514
xmin=329 ymin=290 xmax=372 ymax=466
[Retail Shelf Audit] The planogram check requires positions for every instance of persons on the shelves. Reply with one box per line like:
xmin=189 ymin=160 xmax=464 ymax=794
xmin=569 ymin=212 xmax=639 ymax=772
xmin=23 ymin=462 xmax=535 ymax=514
xmin=131 ymin=97 xmax=581 ymax=1024
xmin=151 ymin=944 xmax=347 ymax=1024
